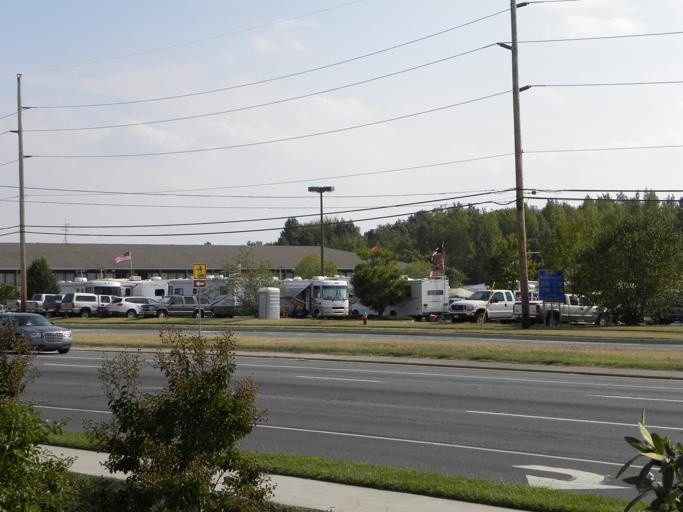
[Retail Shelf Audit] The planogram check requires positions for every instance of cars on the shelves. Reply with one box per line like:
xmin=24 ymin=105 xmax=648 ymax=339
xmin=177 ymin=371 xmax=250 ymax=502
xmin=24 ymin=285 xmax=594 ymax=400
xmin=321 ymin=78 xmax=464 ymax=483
xmin=0 ymin=311 xmax=74 ymax=357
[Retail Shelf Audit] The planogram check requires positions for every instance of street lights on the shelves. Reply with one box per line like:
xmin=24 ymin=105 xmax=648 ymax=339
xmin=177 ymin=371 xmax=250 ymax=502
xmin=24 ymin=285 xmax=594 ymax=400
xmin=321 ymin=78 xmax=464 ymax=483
xmin=306 ymin=185 xmax=335 ymax=277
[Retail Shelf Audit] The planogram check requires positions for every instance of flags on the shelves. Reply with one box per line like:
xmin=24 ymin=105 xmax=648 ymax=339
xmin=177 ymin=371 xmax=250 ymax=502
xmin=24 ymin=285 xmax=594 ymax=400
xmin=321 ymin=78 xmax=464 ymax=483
xmin=114 ymin=252 xmax=129 ymax=263
xmin=370 ymin=245 xmax=377 ymax=251
xmin=429 ymin=258 xmax=444 ymax=278
xmin=431 ymin=244 xmax=442 ymax=259
